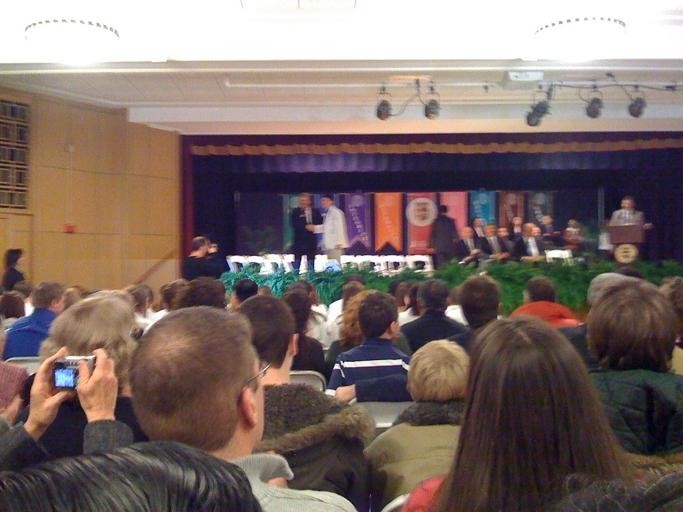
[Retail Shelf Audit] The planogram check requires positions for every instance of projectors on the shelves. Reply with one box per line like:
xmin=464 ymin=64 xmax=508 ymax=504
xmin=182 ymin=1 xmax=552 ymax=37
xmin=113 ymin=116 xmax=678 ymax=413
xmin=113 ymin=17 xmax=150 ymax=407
xmin=502 ymin=71 xmax=544 ymax=90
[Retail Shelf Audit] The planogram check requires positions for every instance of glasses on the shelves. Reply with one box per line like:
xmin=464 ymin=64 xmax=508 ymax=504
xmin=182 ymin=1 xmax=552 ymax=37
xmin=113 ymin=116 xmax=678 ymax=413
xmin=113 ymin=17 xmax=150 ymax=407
xmin=241 ymin=359 xmax=273 ymax=389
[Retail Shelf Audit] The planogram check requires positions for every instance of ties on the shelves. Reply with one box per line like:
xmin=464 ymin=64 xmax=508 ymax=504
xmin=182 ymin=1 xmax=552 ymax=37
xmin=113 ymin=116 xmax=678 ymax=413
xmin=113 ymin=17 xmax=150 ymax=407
xmin=307 ymin=209 xmax=313 ymax=224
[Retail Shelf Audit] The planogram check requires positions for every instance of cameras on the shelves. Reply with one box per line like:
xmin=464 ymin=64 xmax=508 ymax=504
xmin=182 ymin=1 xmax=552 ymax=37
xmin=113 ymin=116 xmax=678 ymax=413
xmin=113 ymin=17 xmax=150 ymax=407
xmin=52 ymin=357 xmax=95 ymax=387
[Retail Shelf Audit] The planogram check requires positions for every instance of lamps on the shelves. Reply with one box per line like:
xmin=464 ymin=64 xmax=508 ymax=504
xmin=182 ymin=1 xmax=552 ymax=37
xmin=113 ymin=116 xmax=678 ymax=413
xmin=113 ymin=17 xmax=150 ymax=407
xmin=523 ymin=79 xmax=649 ymax=129
xmin=372 ymin=78 xmax=452 ymax=128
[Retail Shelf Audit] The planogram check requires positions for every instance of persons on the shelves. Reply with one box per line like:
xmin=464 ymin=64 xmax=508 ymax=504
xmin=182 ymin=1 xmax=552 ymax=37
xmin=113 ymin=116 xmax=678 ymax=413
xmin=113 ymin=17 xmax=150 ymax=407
xmin=1 ymin=193 xmax=683 ymax=512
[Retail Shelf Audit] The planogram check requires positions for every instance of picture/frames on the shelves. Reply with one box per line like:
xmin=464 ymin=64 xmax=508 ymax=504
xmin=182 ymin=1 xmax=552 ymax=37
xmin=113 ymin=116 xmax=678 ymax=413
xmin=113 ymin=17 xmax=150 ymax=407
xmin=0 ymin=91 xmax=38 ymax=216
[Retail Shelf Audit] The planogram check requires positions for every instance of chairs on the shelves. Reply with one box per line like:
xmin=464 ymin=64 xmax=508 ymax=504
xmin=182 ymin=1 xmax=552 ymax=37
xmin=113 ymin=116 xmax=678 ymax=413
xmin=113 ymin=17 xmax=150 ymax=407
xmin=0 ymin=354 xmax=428 ymax=512
xmin=223 ymin=245 xmax=574 ymax=276
